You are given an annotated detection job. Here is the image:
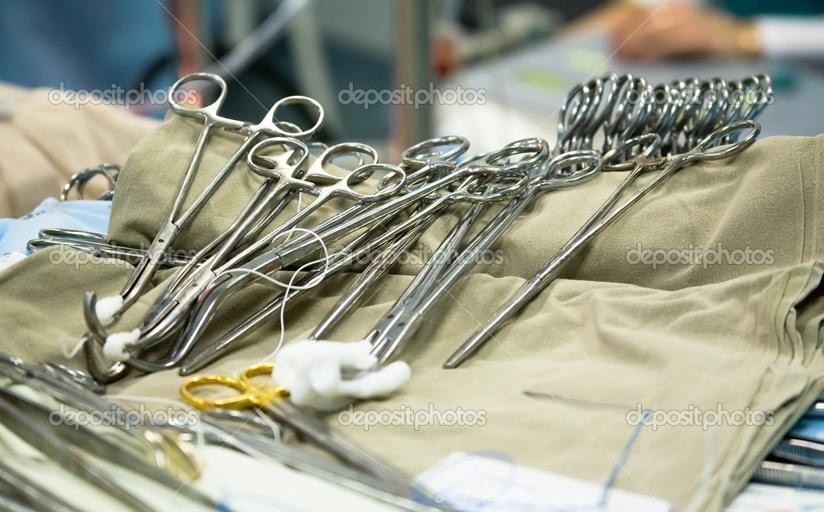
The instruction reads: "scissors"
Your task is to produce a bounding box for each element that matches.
[60,66,775,388]
[0,349,470,512]
